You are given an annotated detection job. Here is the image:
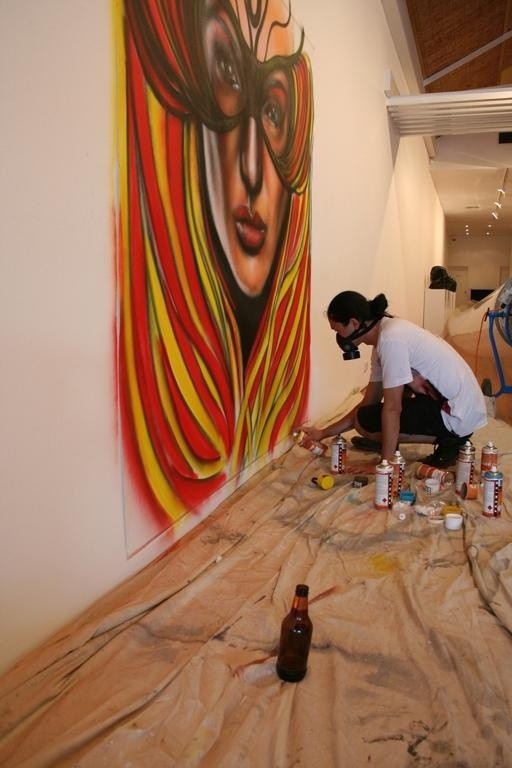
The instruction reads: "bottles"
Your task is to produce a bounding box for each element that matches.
[273,583,315,686]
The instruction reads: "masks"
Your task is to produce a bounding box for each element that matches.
[336,330,361,352]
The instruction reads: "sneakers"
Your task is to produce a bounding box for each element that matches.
[351,436,400,451]
[424,434,473,468]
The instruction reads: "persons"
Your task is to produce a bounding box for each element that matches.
[111,0,311,534]
[289,290,489,470]
